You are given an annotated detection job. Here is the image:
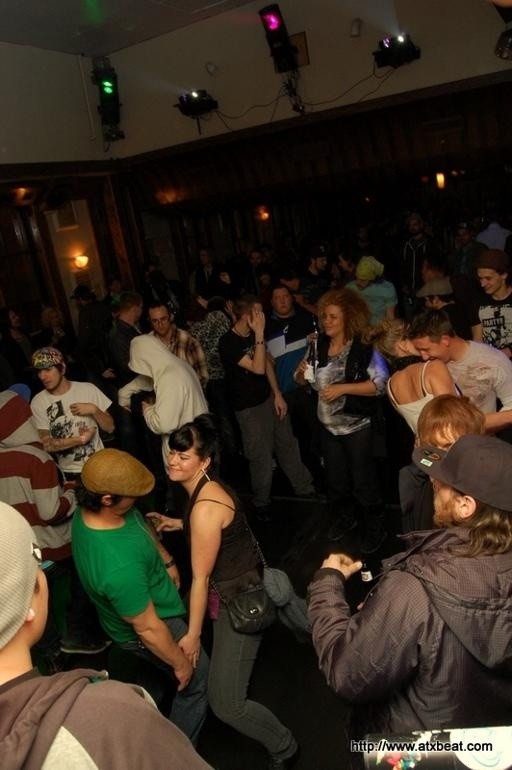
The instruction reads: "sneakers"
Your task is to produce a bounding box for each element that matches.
[360,528,388,553]
[328,513,357,540]
[267,742,301,770]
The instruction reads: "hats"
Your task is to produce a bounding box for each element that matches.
[81,448,155,497]
[0,503,34,651]
[31,347,62,369]
[475,249,509,274]
[416,277,453,298]
[413,433,512,513]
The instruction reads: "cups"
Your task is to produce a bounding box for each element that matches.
[149,518,163,540]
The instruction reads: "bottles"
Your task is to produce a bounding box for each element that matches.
[304,343,319,384]
[312,320,319,342]
[360,560,375,592]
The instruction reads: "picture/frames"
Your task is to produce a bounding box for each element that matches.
[49,204,81,233]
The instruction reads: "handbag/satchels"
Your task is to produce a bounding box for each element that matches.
[224,585,278,633]
